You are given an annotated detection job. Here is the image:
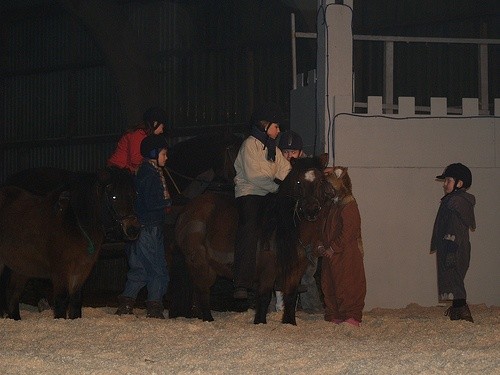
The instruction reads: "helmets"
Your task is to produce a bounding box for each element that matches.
[279,131,303,151]
[140,135,168,157]
[436,164,472,189]
[255,102,285,123]
[143,108,169,125]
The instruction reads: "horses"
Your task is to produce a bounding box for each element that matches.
[0,176,143,320]
[175,154,329,327]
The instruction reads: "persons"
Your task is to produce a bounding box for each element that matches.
[106,106,168,169]
[430,163,476,321]
[234,119,292,298]
[116,136,175,318]
[275,131,321,312]
[317,167,366,326]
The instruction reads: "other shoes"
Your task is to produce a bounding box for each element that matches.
[443,306,473,323]
[341,319,358,327]
[116,304,132,315]
[331,319,339,325]
[234,288,248,299]
[147,304,164,319]
